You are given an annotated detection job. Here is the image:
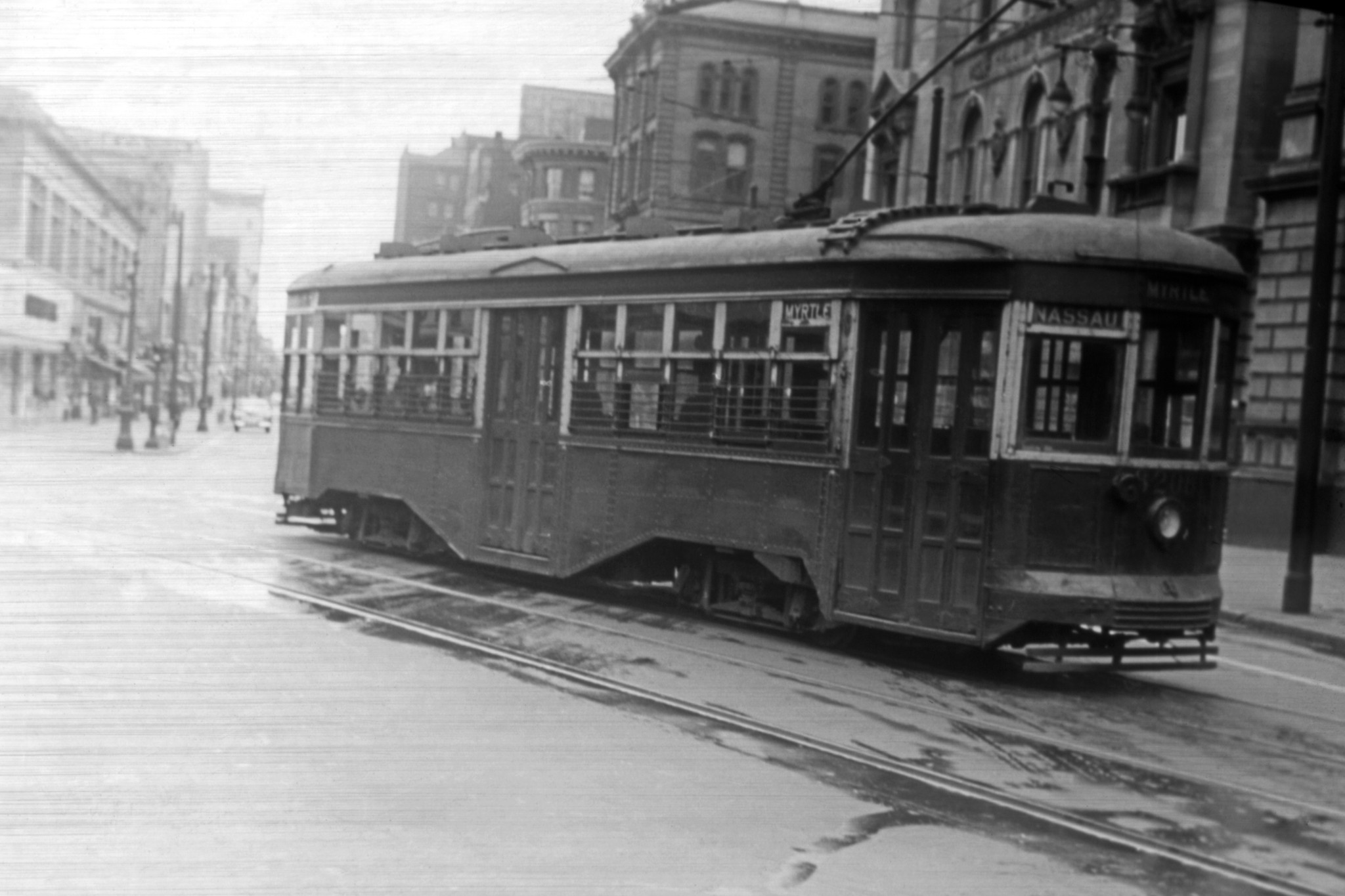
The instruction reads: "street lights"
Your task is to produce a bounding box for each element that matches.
[1047,42,1164,210]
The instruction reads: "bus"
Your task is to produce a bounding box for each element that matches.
[273,201,1258,676]
